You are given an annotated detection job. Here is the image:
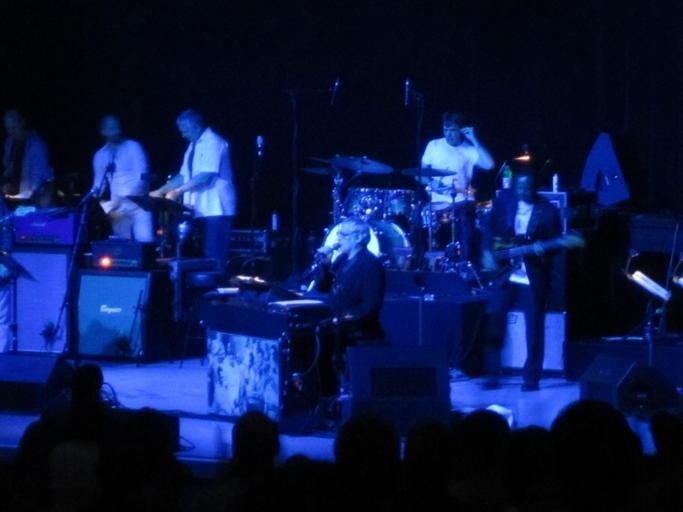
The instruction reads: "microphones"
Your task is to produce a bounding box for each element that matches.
[312,243,341,263]
[255,136,265,156]
[329,79,341,105]
[108,143,117,175]
[401,78,411,107]
[204,286,239,298]
[178,204,194,239]
[77,184,98,207]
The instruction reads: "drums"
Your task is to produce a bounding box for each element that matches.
[420,201,451,229]
[346,187,388,222]
[321,223,411,279]
[381,188,417,218]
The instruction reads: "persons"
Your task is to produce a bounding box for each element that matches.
[149,108,237,274]
[86,114,153,242]
[479,172,561,393]
[421,110,498,258]
[0,108,57,209]
[1,398,682,512]
[299,219,386,396]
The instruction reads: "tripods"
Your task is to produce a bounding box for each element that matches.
[415,178,485,293]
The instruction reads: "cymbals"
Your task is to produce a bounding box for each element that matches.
[334,155,393,174]
[402,168,456,178]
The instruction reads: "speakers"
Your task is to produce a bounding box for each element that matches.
[11,245,76,356]
[614,360,682,420]
[73,267,172,363]
[0,350,76,411]
[88,238,155,271]
[12,207,86,245]
[341,346,452,428]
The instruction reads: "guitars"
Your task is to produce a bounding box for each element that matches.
[476,230,587,279]
